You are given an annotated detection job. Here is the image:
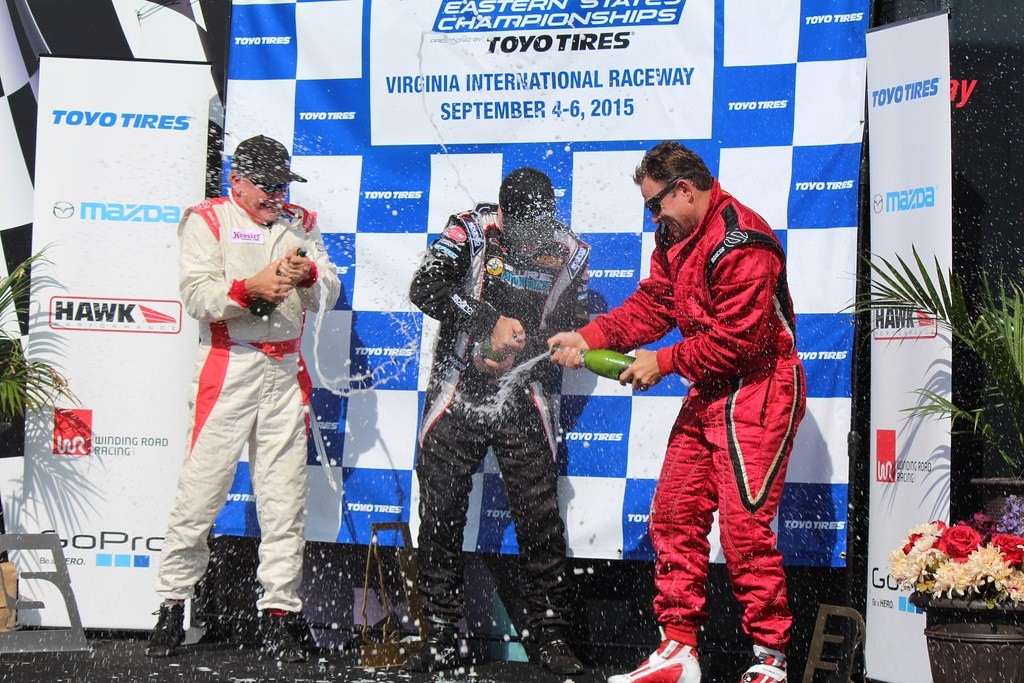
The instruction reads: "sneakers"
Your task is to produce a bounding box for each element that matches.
[528,625,584,675]
[400,623,462,670]
[258,613,305,662]
[741,643,788,683]
[605,626,701,683]
[148,605,185,656]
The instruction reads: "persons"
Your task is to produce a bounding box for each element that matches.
[143,134,342,661]
[410,169,591,676]
[546,140,807,683]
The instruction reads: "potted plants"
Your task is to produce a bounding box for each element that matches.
[837,243,1024,531]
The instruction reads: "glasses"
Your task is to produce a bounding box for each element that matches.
[645,175,684,216]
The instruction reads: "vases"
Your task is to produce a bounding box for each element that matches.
[907,589,1023,682]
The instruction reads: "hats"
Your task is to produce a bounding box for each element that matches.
[499,168,555,243]
[230,135,308,192]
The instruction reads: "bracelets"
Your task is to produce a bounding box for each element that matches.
[641,386,646,391]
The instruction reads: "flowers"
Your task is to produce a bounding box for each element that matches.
[887,495,1023,610]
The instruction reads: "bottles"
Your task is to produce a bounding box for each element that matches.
[249,248,307,317]
[551,346,637,384]
[471,334,523,363]
